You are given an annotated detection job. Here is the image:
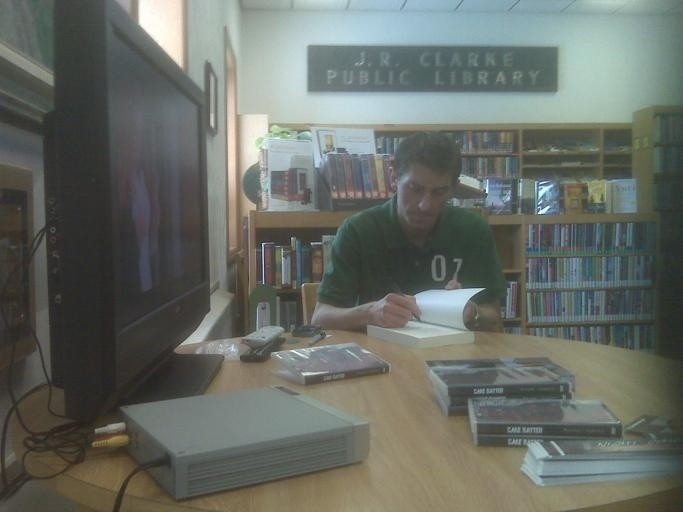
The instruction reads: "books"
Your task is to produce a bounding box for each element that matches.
[366,288,485,347]
[447,129,519,215]
[261,136,405,211]
[258,235,335,329]
[654,115,681,177]
[522,140,626,153]
[501,280,521,335]
[519,179,655,352]
[423,353,628,449]
[272,342,391,386]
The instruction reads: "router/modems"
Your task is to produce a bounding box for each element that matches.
[117,384,370,500]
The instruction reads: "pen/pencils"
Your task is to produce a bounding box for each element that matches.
[310,332,326,345]
[393,283,422,322]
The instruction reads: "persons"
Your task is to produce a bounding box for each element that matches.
[309,133,509,332]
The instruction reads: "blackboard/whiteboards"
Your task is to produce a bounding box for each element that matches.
[308,45,557,92]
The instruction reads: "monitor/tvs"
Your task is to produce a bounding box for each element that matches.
[43,1,223,421]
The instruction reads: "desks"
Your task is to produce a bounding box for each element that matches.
[24,328,683,512]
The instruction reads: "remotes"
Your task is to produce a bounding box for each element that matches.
[240,325,283,348]
[241,337,286,362]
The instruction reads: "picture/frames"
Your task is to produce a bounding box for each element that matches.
[201,62,220,135]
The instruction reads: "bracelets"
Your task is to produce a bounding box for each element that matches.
[472,306,479,327]
